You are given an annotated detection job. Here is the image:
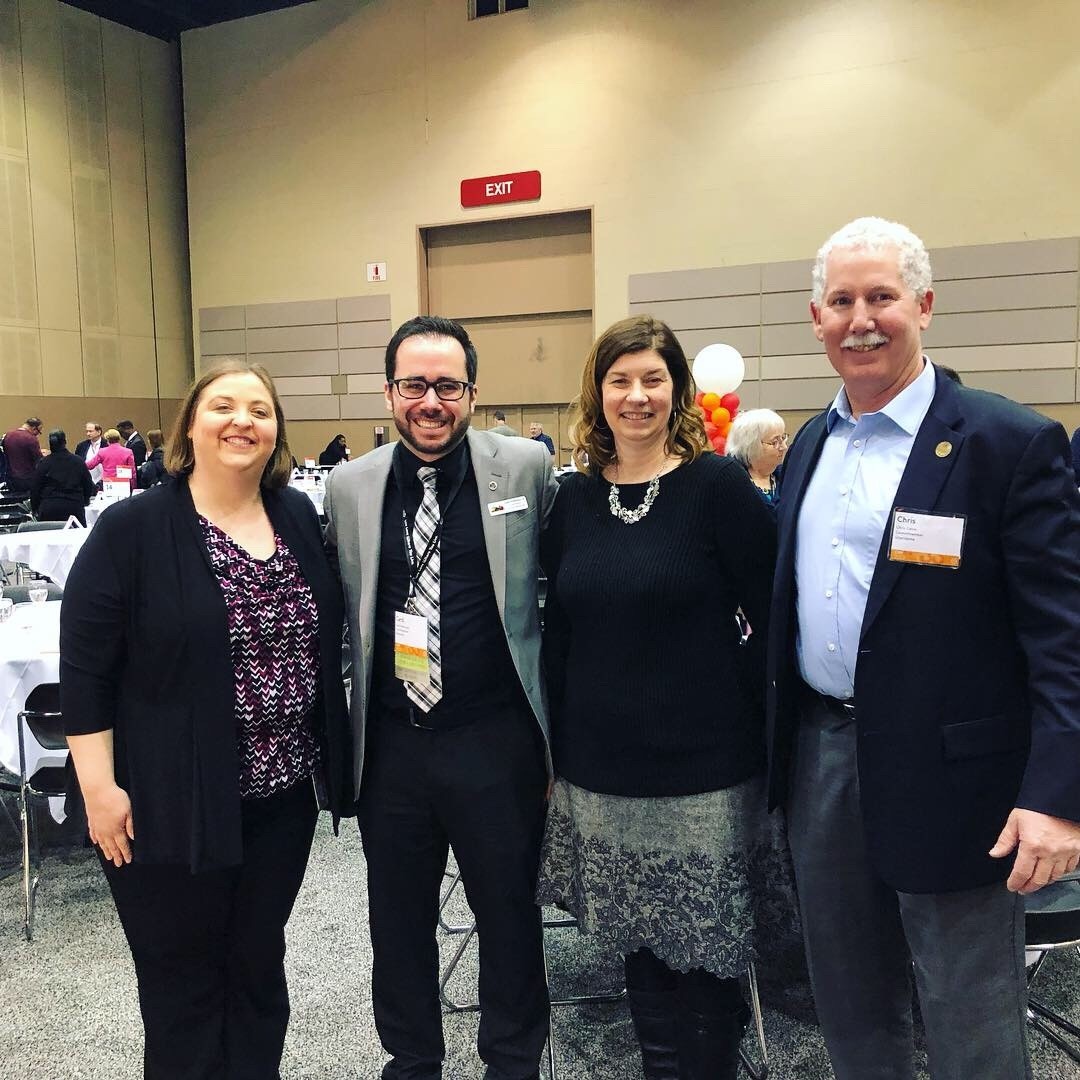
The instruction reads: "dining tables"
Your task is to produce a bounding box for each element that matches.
[0,600,72,792]
[85,499,126,526]
[291,480,328,514]
[0,526,96,590]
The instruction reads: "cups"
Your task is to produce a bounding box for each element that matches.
[0,598,13,624]
[29,590,48,606]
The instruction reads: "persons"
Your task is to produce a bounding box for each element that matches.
[533,313,803,1080]
[58,360,355,1080]
[116,421,147,467]
[489,411,517,437]
[146,429,167,473]
[529,423,555,462]
[723,409,788,506]
[325,316,562,1080]
[3,417,45,494]
[85,428,137,490]
[319,434,353,470]
[31,430,92,529]
[76,423,109,491]
[759,217,1080,1080]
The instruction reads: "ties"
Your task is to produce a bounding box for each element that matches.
[406,467,441,713]
[92,442,98,455]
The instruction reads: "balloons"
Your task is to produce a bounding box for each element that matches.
[691,344,744,399]
[693,393,740,456]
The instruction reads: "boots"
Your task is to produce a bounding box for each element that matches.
[631,999,741,1079]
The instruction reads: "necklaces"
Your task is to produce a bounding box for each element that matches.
[608,455,668,524]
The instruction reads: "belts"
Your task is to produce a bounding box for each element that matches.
[799,682,855,718]
[377,705,498,730]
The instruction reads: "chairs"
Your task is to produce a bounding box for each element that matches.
[17,683,82,943]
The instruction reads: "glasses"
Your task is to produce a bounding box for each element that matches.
[389,378,473,402]
[36,429,42,435]
[762,433,790,448]
